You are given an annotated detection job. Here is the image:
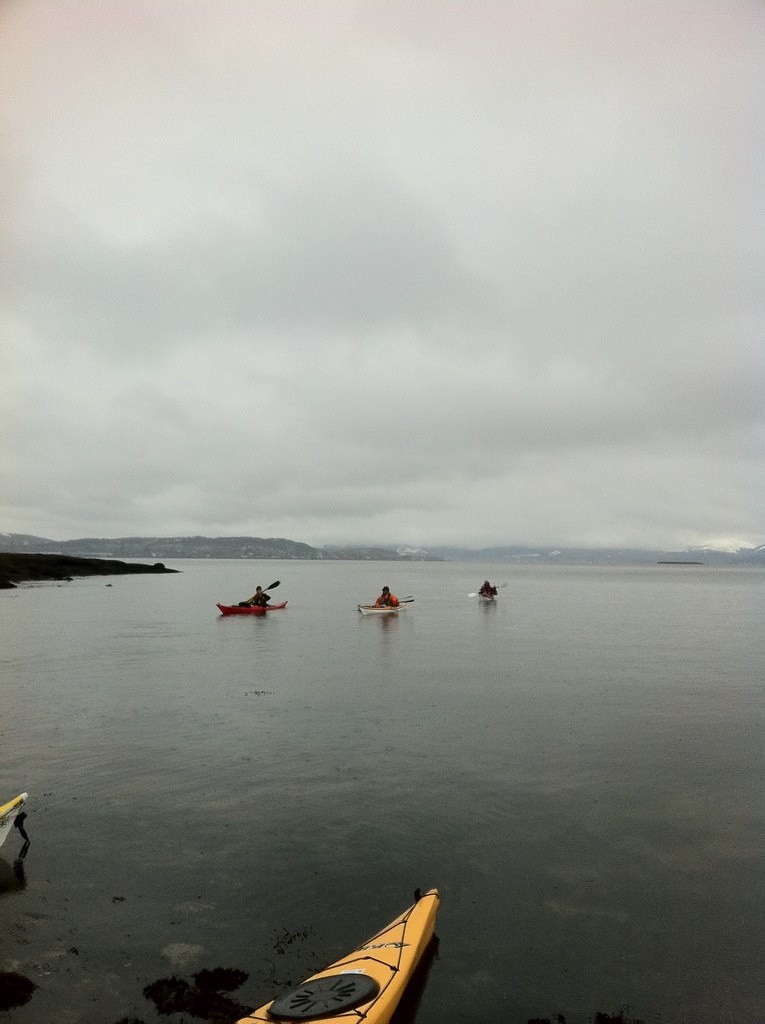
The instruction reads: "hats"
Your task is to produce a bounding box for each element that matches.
[382,586,389,591]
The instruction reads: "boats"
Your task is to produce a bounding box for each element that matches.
[235,887,440,1024]
[0,792,30,848]
[358,604,407,615]
[479,589,499,602]
[216,601,288,615]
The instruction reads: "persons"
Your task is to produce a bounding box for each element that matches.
[375,586,399,606]
[480,581,497,595]
[252,586,271,606]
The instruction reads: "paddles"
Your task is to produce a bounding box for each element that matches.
[357,599,415,612]
[246,581,280,602]
